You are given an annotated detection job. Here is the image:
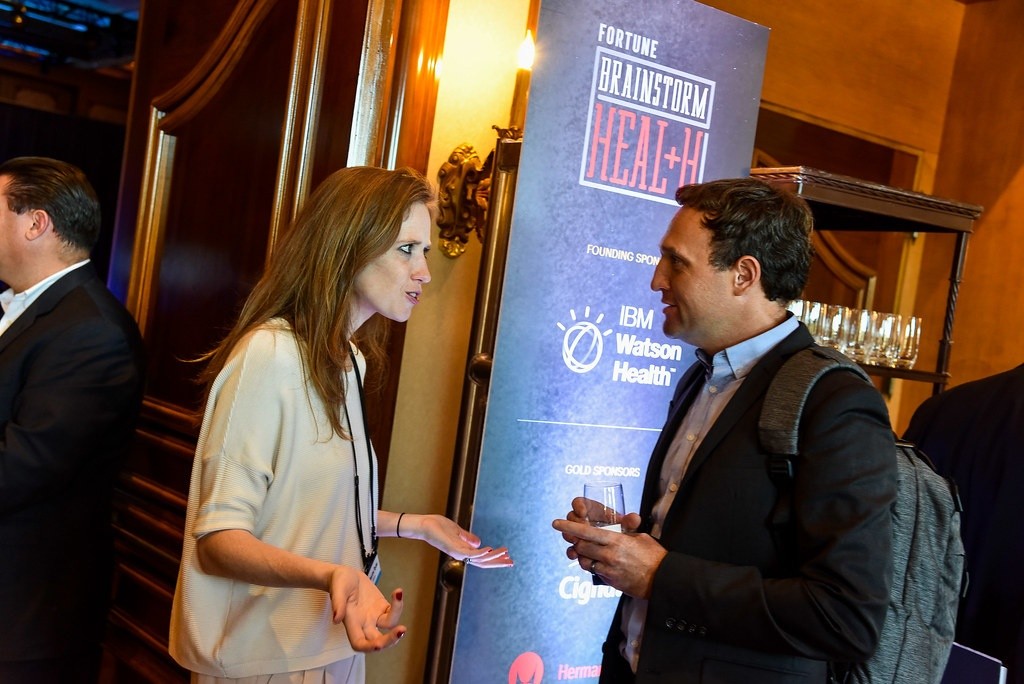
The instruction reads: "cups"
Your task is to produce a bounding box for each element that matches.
[584,481,628,534]
[784,298,923,370]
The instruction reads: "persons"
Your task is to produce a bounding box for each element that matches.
[0,157,151,684]
[900,364,1024,684]
[169,165,515,684]
[552,177,901,684]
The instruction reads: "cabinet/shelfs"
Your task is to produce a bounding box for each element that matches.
[750,165,985,397]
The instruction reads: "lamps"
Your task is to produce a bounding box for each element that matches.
[435,28,537,259]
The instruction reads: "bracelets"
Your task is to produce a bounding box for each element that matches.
[396,513,406,538]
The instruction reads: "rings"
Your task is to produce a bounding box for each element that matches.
[590,560,599,573]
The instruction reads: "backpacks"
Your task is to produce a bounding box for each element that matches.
[759,343,967,684]
